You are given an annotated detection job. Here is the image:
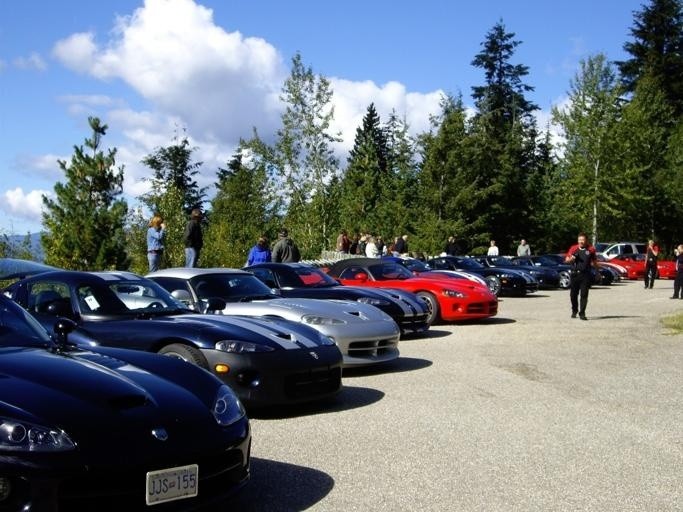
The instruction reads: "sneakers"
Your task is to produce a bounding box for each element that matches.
[670,296,679,299]
[571,310,577,318]
[644,285,648,289]
[579,312,587,320]
[650,285,653,289]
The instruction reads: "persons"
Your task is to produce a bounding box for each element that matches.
[564,232,597,320]
[146,215,166,272]
[445,237,457,256]
[269,227,300,264]
[674,246,680,272]
[670,245,683,299]
[246,236,271,267]
[486,240,499,256]
[181,209,202,268]
[337,224,409,261]
[643,240,659,289]
[517,239,531,256]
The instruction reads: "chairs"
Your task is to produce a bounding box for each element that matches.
[35,290,61,306]
